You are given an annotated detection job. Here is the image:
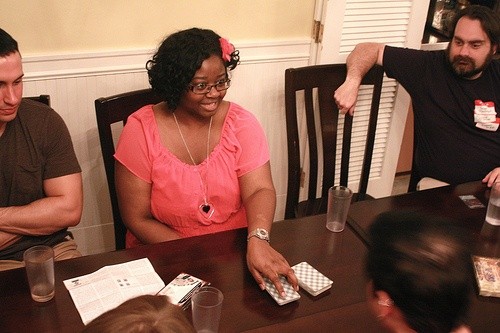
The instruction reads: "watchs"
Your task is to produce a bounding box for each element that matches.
[246,227,270,244]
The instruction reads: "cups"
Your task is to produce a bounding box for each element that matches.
[23,245,55,303]
[485,182,500,226]
[326,186,353,232]
[191,287,224,333]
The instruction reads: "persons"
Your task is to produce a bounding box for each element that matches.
[0,27,84,271]
[112,29,299,297]
[363,206,474,333]
[332,6,500,191]
[79,295,196,333]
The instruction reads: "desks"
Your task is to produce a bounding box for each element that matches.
[0,181,500,333]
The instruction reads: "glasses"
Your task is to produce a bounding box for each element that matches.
[186,78,231,95]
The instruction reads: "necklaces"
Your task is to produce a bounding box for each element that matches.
[170,108,214,219]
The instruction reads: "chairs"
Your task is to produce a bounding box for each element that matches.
[95,89,166,250]
[285,64,384,220]
